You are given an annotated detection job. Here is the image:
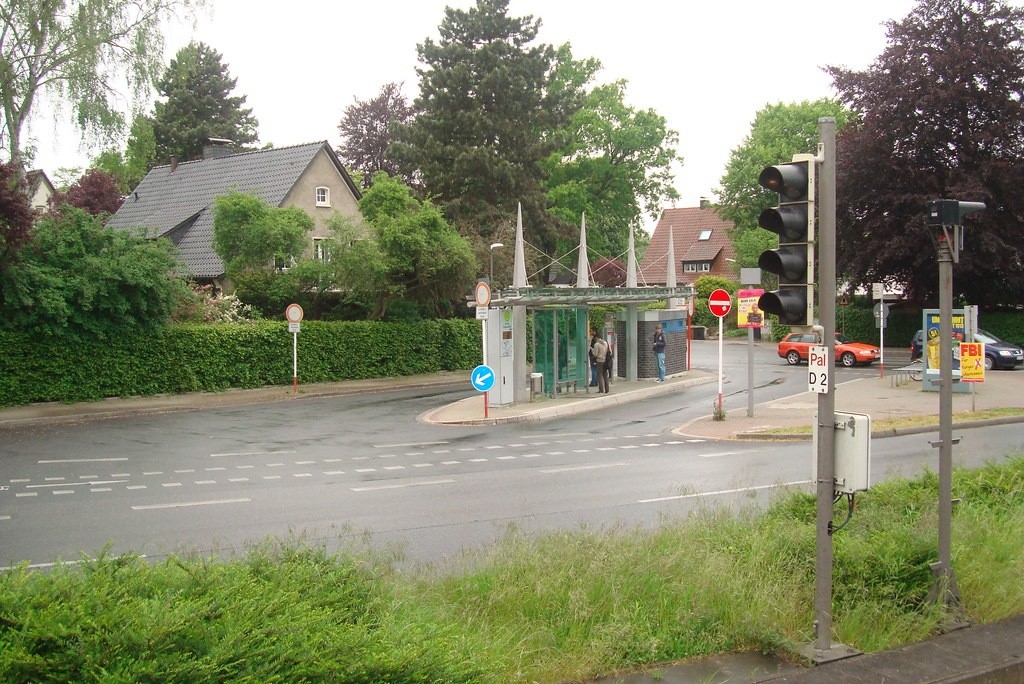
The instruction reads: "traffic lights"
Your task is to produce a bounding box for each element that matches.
[757,154,815,326]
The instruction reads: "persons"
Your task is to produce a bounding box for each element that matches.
[558,329,567,393]
[652,324,666,383]
[747,304,763,321]
[590,334,612,394]
[589,326,602,387]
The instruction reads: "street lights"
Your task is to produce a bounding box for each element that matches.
[489,242,504,284]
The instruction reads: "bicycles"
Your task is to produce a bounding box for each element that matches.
[908,358,923,381]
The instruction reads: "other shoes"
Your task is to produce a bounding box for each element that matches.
[656,378,664,383]
[595,391,604,394]
[589,383,598,387]
[605,391,609,394]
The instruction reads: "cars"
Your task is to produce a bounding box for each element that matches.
[777,331,881,367]
[911,327,1024,370]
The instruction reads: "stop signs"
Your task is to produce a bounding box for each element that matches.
[708,289,731,317]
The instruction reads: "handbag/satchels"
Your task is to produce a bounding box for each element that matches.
[604,340,612,369]
